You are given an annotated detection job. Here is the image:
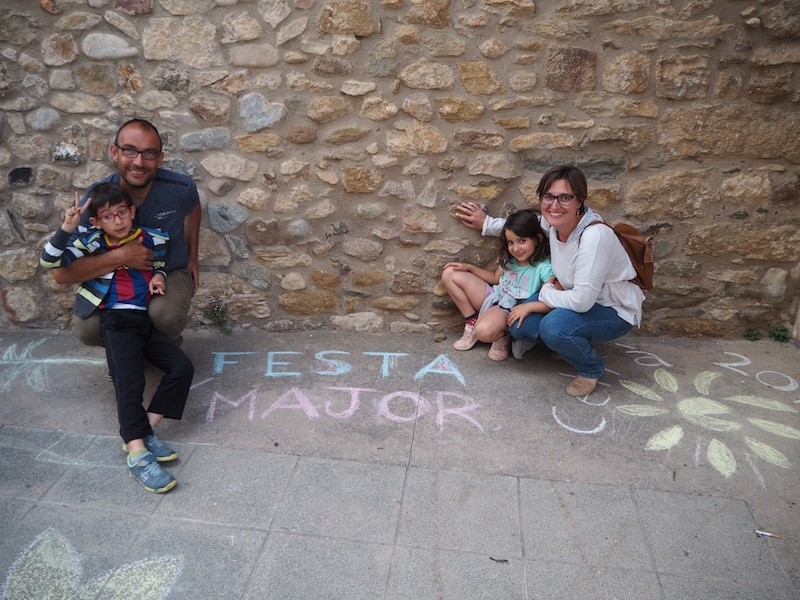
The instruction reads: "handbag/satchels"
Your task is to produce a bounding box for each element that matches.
[577,221,654,290]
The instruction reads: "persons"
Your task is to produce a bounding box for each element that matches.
[52,119,202,381]
[441,210,562,362]
[39,181,194,493]
[455,165,646,396]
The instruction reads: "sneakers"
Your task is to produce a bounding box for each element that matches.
[126,452,178,494]
[488,335,511,361]
[123,431,178,462]
[454,322,479,351]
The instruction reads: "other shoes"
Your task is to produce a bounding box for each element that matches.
[107,369,113,381]
[565,375,598,397]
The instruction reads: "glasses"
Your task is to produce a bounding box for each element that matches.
[540,193,575,205]
[115,142,161,160]
[95,205,131,224]
